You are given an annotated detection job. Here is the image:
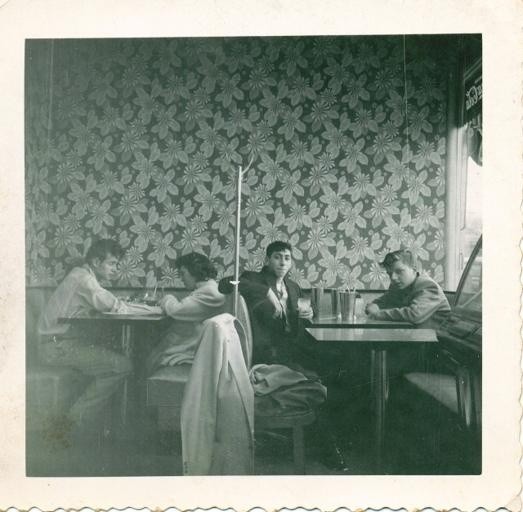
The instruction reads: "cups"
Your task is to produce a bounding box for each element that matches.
[310,286,324,314]
[331,288,356,322]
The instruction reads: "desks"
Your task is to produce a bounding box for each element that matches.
[55,306,169,475]
[305,309,438,473]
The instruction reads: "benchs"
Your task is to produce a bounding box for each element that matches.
[147,290,316,476]
[24,294,84,475]
[401,360,481,475]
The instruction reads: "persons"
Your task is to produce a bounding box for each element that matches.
[219,242,355,472]
[363,249,456,375]
[134,253,232,449]
[33,238,135,451]
